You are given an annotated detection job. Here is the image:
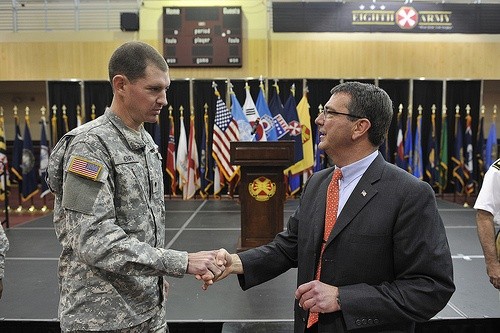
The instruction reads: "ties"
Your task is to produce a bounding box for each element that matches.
[307,168,342,328]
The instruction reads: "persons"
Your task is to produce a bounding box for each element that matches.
[473,158,500,290]
[44,40,227,333]
[203,82,456,333]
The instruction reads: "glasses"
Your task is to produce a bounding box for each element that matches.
[324,108,364,120]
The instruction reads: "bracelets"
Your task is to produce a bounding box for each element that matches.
[336,294,341,306]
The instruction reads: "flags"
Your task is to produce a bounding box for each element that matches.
[380,114,497,197]
[207,82,333,198]
[151,111,213,201]
[0,113,95,201]
